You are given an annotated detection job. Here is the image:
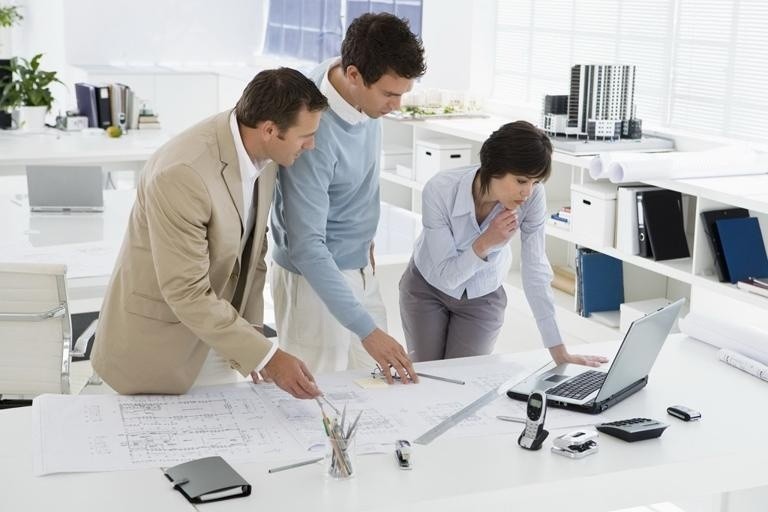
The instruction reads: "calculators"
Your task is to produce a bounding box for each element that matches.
[596,418,668,441]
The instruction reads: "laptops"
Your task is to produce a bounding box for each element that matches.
[505,295,687,415]
[25,164,106,213]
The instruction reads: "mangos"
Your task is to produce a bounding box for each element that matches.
[107,126,122,137]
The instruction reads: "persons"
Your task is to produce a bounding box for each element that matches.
[268,12,427,386]
[86,64,329,400]
[397,119,610,368]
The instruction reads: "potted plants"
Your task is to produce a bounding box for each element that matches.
[0,51,70,133]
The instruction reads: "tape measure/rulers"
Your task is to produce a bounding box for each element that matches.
[413,356,554,446]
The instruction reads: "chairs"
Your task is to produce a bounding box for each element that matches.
[0,260,98,400]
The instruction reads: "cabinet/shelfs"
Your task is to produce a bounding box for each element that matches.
[376,111,767,332]
[72,60,259,128]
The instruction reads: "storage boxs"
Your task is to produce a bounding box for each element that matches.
[380,137,471,186]
[569,182,688,247]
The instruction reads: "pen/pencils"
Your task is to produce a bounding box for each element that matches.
[316,395,342,417]
[416,372,465,385]
[269,458,326,473]
[322,404,364,477]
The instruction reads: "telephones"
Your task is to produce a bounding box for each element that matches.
[518,391,548,451]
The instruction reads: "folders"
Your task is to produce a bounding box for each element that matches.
[636,190,689,257]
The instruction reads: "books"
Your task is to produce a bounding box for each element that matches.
[164,455,251,505]
[75,82,161,133]
[616,184,768,299]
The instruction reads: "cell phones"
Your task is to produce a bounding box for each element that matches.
[667,405,702,422]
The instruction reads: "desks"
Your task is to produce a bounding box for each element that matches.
[1,333,766,512]
